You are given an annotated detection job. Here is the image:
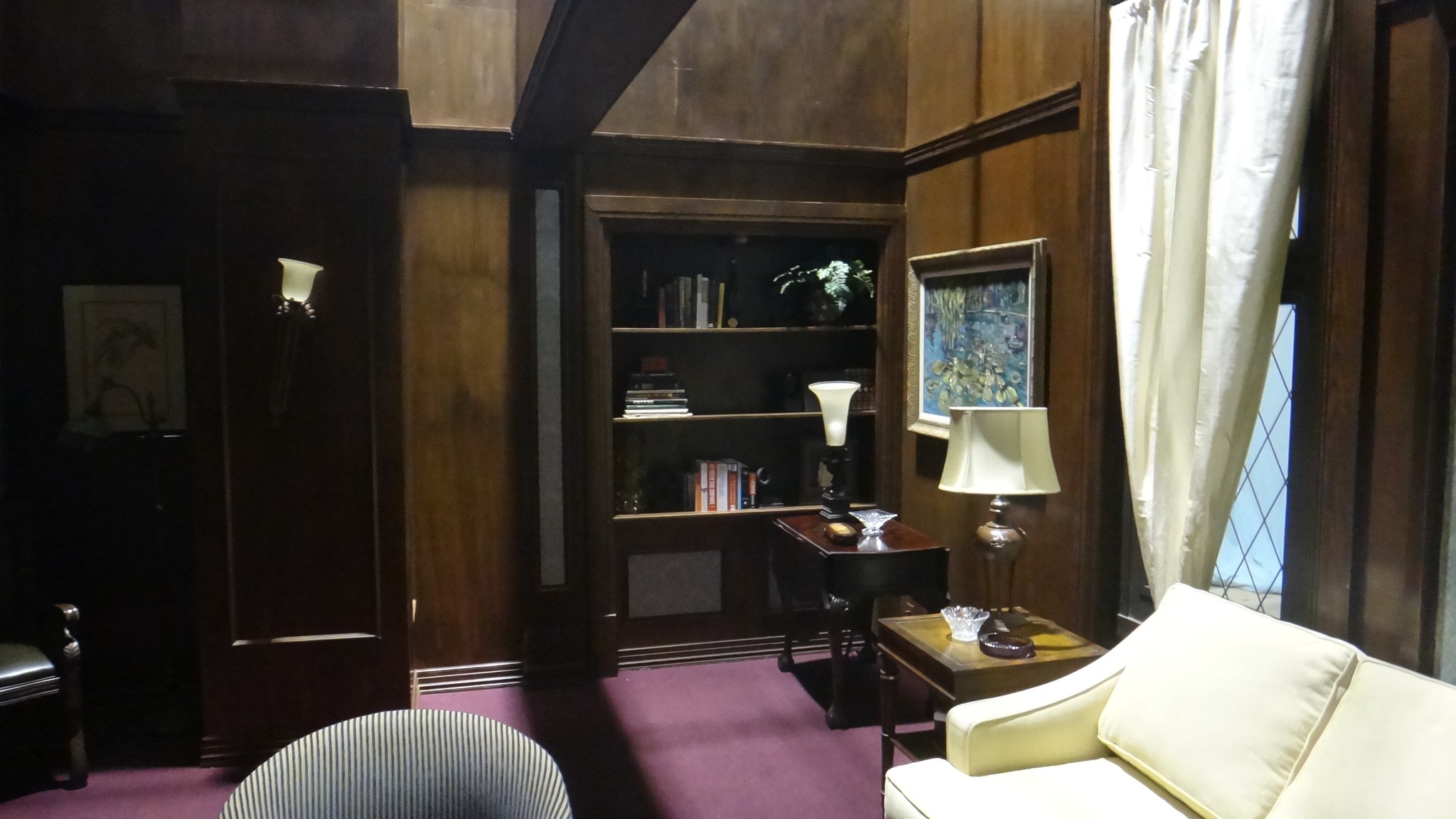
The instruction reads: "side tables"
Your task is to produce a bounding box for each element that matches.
[877,606,1111,813]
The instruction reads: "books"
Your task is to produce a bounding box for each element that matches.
[619,352,693,417]
[657,272,725,329]
[681,456,756,513]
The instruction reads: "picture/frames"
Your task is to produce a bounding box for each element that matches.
[63,285,187,437]
[905,236,1048,443]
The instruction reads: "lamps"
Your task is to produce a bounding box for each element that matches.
[937,405,1062,635]
[806,380,862,522]
[266,254,324,424]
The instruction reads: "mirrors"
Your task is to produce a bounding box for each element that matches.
[218,147,387,647]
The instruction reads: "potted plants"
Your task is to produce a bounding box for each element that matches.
[773,251,875,325]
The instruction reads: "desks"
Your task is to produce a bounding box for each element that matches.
[770,512,950,730]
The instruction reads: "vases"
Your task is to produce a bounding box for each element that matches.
[619,434,650,513]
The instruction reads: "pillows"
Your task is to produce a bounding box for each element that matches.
[1265,658,1456,819]
[1098,583,1358,819]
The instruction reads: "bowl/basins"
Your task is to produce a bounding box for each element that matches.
[941,605,990,641]
[849,509,898,535]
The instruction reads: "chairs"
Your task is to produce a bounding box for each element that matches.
[0,595,90,793]
[218,707,575,819]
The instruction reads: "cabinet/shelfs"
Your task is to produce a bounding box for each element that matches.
[584,193,911,664]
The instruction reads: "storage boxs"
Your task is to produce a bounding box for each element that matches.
[825,522,859,545]
[636,355,667,373]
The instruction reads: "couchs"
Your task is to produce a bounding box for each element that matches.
[884,583,1456,819]
